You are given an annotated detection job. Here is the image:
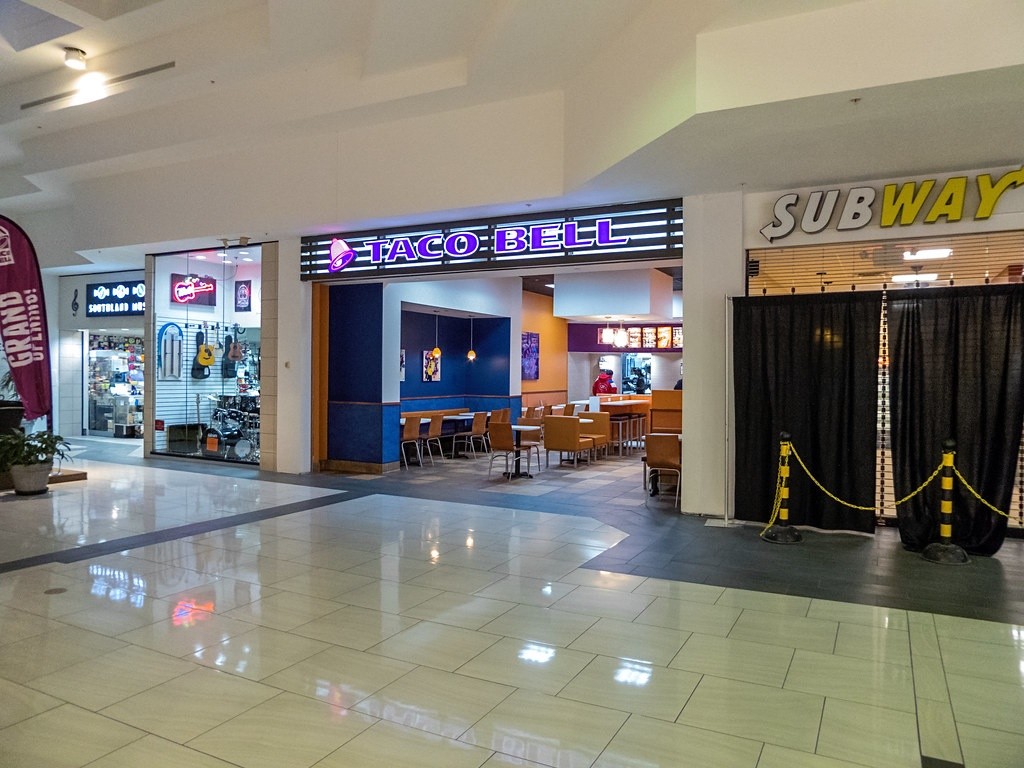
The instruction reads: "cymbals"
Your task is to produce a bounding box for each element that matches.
[204,392,221,402]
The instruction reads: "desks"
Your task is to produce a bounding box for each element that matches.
[400,418,431,466]
[503,425,540,479]
[561,419,593,465]
[442,416,473,459]
[599,399,649,405]
[459,411,492,417]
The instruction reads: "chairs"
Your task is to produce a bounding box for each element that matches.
[400,393,682,509]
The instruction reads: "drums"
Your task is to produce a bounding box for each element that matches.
[211,407,226,422]
[234,438,256,459]
[218,394,239,410]
[241,413,260,432]
[239,395,260,414]
[226,409,243,425]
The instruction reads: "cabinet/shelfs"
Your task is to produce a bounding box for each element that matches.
[113,395,144,438]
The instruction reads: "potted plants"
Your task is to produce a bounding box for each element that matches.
[0,428,73,496]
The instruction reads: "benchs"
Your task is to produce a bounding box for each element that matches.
[400,408,470,454]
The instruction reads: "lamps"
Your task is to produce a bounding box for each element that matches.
[433,309,442,358]
[467,315,476,361]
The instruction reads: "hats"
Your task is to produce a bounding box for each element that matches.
[642,365,651,369]
[634,367,642,371]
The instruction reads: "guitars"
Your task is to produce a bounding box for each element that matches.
[194,394,203,449]
[197,320,217,366]
[229,324,242,360]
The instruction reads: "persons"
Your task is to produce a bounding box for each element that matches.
[592,370,617,397]
[131,384,142,395]
[673,378,682,391]
[634,368,646,394]
